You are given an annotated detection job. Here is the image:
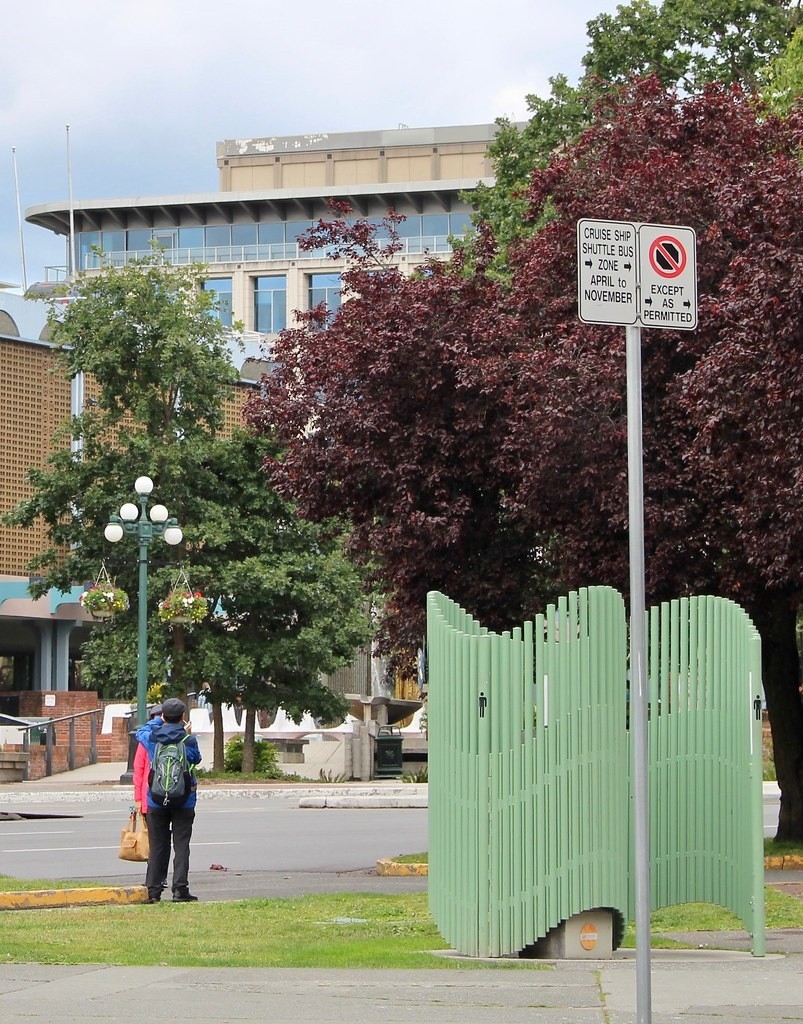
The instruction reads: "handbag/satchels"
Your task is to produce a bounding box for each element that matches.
[118,810,149,861]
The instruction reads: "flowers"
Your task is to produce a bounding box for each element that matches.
[156,588,207,633]
[79,583,130,622]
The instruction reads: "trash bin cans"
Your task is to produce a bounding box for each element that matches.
[376,724,405,775]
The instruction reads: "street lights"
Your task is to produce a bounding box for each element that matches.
[103,476,184,786]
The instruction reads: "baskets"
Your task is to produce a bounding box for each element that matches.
[92,567,115,616]
[168,572,194,623]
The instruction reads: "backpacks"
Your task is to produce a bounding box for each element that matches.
[148,734,195,806]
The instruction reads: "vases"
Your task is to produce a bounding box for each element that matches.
[92,609,115,617]
[170,616,192,623]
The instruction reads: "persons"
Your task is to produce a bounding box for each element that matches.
[135,698,202,904]
[132,705,172,888]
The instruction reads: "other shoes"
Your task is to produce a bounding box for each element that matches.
[173,894,198,903]
[143,897,160,904]
[160,879,168,888]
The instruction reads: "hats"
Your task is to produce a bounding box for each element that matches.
[150,704,162,716]
[162,698,185,716]
